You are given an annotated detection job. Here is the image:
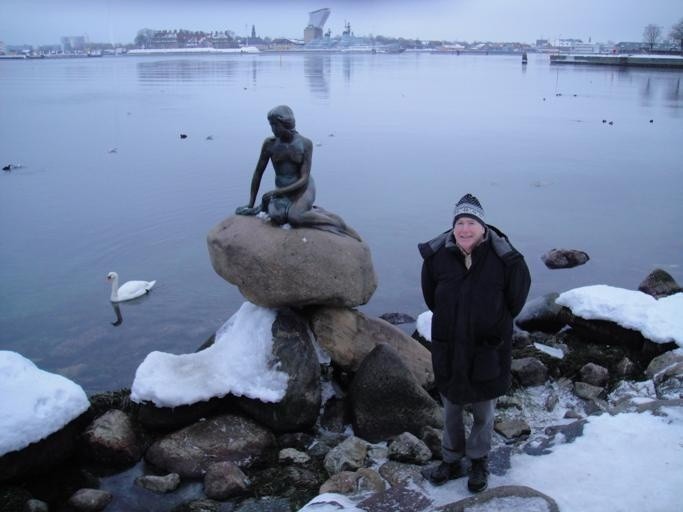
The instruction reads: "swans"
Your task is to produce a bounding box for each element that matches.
[108,291,155,328]
[104,271,157,305]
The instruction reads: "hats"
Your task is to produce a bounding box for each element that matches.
[452,192,486,229]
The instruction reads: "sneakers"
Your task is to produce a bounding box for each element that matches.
[468,456,488,492]
[429,460,461,486]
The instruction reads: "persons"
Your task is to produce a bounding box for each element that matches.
[235,106,362,238]
[417,193,531,494]
